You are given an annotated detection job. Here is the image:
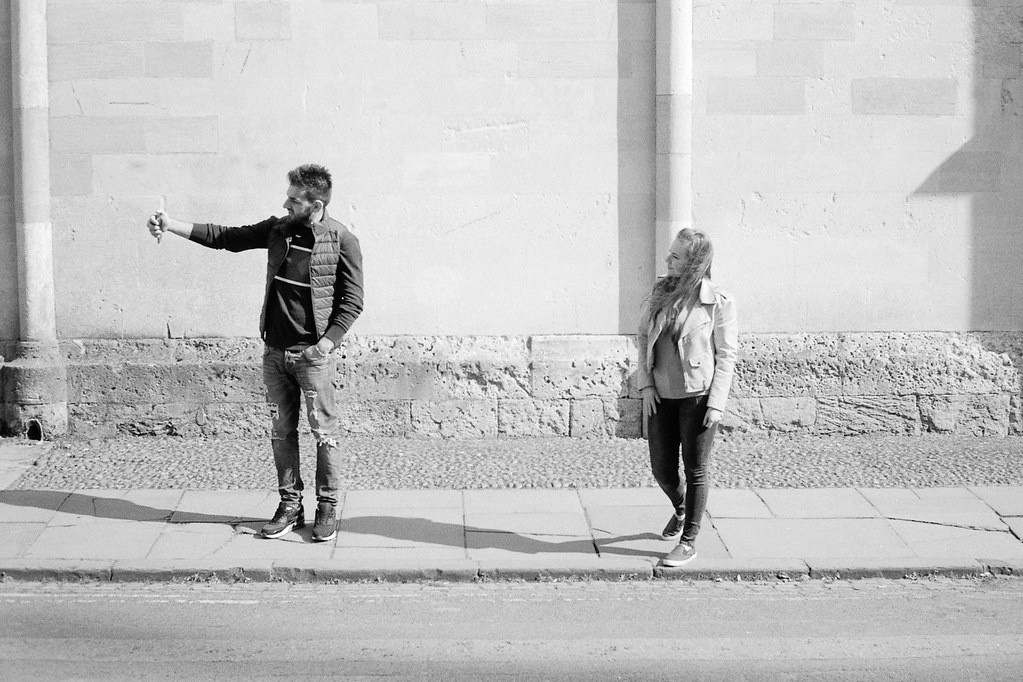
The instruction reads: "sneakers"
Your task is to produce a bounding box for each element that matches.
[261,503,304,538]
[312,503,337,541]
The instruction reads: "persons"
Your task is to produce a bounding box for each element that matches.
[633,227,737,567]
[147,164,364,542]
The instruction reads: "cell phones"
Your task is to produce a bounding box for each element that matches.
[155,213,163,243]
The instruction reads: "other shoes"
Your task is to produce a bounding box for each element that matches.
[663,545,697,567]
[662,514,686,539]
